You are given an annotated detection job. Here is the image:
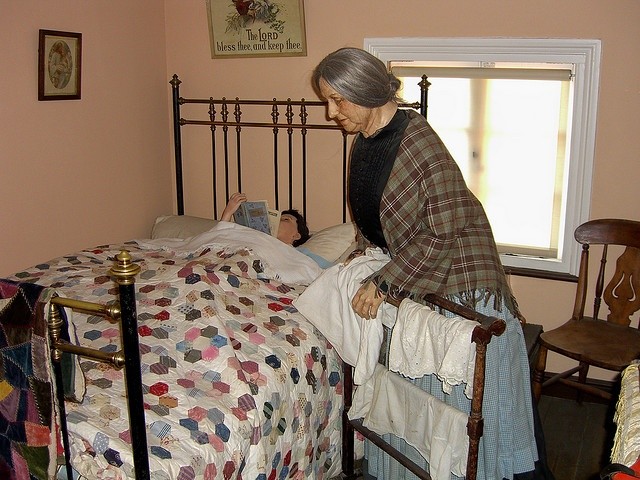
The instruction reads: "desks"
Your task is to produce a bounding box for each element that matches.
[521,323,544,361]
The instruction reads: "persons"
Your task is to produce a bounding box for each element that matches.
[217,189,310,247]
[312,48,539,480]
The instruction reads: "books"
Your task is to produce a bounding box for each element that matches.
[232,198,280,241]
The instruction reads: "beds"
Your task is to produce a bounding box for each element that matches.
[0,72,432,480]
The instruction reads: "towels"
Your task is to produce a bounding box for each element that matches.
[0,278,86,480]
[346,364,471,478]
[291,248,399,385]
[389,298,481,400]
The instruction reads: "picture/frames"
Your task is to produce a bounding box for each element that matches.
[37,30,82,102]
[205,1,308,59]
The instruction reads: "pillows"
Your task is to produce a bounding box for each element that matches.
[610,358,640,473]
[151,215,358,267]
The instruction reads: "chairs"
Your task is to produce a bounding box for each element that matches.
[532,218,640,404]
